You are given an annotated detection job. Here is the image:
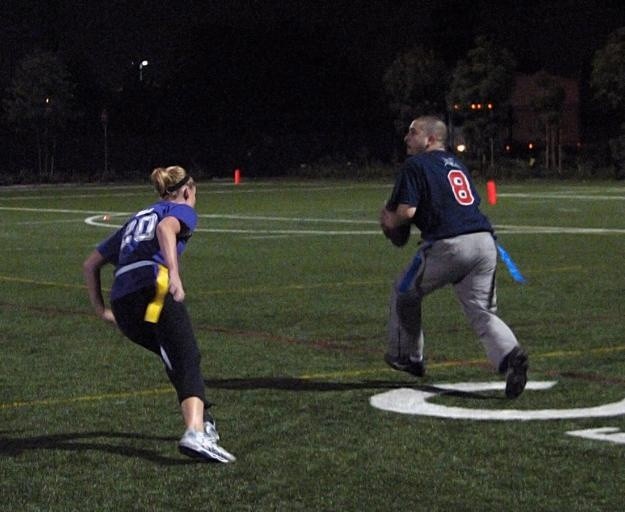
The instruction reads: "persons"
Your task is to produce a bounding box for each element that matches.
[82,164,240,466]
[378,114,533,400]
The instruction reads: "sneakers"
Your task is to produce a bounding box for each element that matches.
[177,428,237,464]
[506,353,529,399]
[383,351,426,377]
[203,420,222,443]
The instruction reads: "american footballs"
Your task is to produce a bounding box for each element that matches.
[392,222,412,246]
